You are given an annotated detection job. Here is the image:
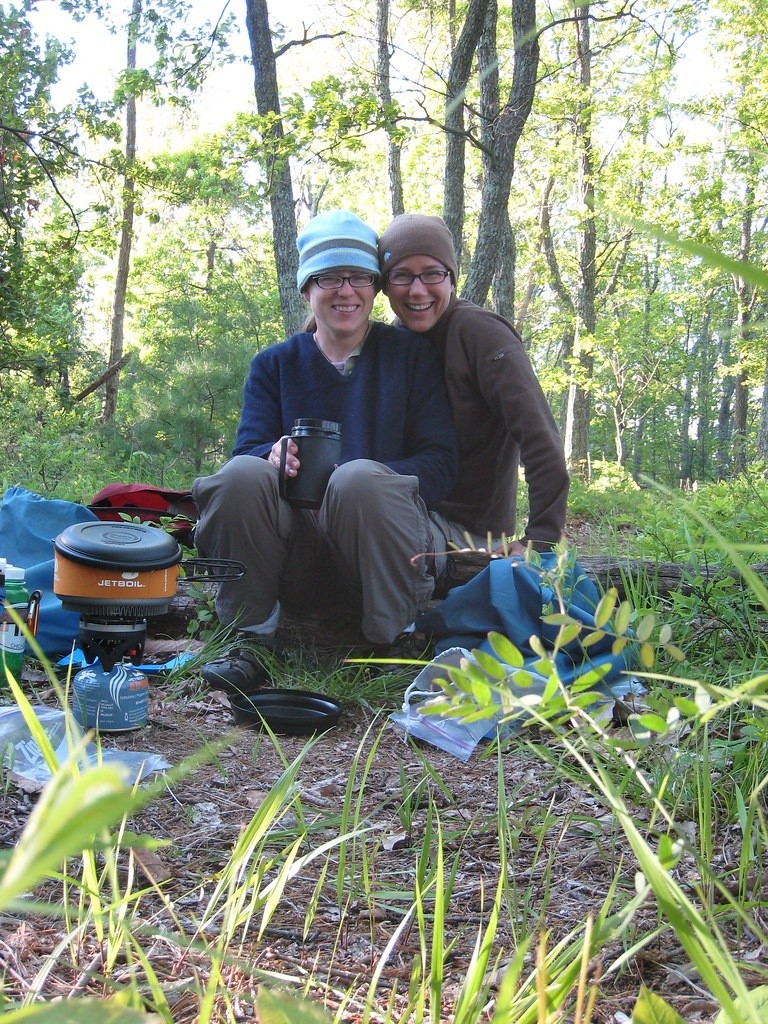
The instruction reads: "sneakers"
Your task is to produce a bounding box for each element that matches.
[375,634,423,683]
[200,636,278,693]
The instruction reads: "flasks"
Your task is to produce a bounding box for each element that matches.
[279,418,343,510]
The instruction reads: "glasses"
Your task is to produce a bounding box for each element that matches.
[384,270,452,286]
[311,272,379,289]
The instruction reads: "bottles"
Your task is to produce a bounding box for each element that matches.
[0,568,43,688]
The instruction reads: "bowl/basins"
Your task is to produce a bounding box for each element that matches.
[232,690,342,734]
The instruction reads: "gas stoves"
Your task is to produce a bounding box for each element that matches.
[71,611,148,733]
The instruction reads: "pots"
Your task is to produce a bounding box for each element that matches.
[52,521,248,607]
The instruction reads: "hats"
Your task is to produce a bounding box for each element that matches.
[377,214,458,289]
[295,210,383,295]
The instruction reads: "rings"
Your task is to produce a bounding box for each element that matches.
[275,457,279,465]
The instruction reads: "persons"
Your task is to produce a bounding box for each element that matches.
[191,210,462,691]
[378,214,570,580]
